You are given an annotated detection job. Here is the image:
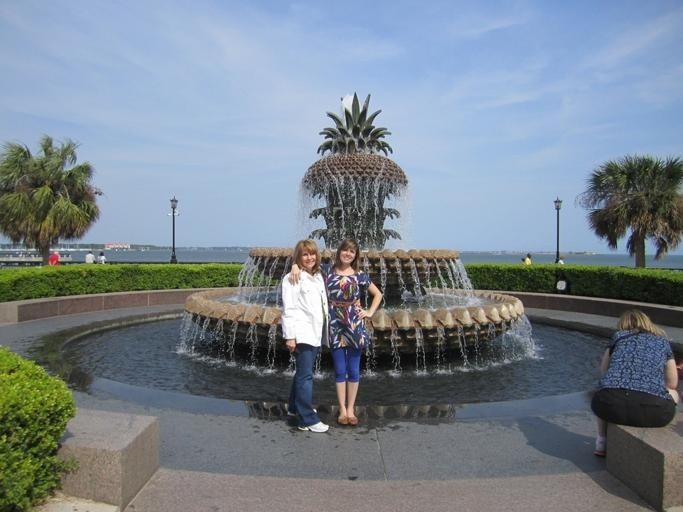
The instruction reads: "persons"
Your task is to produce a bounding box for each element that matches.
[591,309,678,457]
[523,253,532,265]
[86,251,96,264]
[558,256,565,264]
[48,251,59,266]
[289,238,382,426]
[97,251,106,264]
[281,240,330,432]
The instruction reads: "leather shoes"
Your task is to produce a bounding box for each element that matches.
[338,416,358,425]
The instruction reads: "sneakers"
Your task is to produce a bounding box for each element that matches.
[288,409,329,432]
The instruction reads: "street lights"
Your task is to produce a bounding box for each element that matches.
[553,197,562,263]
[170,195,177,264]
